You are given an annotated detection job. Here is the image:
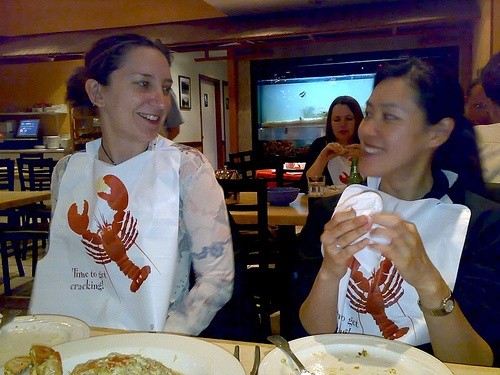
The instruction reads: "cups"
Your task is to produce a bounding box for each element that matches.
[307,175,326,197]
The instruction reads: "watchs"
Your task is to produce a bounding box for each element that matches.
[417,291,455,317]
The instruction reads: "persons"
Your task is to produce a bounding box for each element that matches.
[464,53,500,202]
[28,33,235,338]
[299,97,365,193]
[278,52,500,369]
[164,90,184,141]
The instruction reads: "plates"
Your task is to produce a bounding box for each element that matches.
[34,146,45,149]
[256,334,453,375]
[52,332,248,375]
[43,135,60,148]
[0,313,91,375]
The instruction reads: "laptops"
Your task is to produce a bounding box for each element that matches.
[5,118,42,141]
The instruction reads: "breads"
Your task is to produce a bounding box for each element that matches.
[70,352,174,375]
[4,345,64,375]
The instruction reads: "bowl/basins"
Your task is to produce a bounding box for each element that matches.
[61,138,70,148]
[267,187,301,206]
[32,108,43,112]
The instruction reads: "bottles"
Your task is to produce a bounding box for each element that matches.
[346,162,365,187]
[8,130,14,137]
[0,133,5,143]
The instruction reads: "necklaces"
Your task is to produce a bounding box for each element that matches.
[101,139,115,165]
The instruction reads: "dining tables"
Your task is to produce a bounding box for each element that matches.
[228,189,309,341]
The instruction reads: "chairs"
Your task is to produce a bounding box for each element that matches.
[0,147,342,345]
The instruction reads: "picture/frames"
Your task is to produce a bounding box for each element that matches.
[178,75,192,110]
